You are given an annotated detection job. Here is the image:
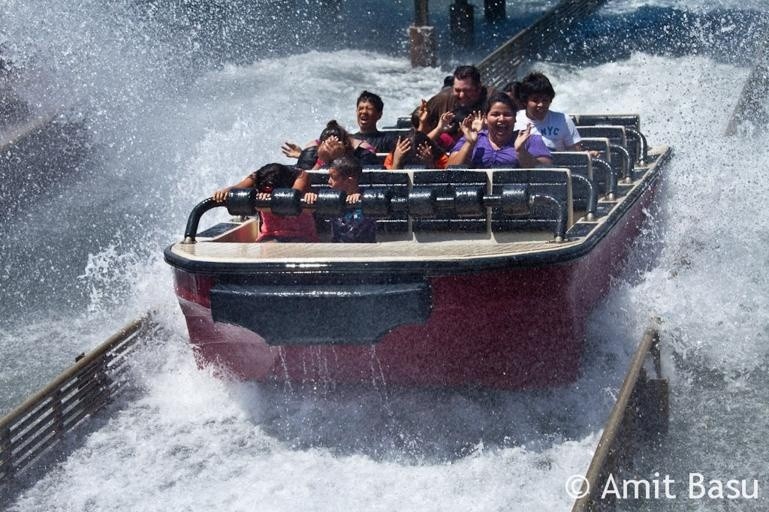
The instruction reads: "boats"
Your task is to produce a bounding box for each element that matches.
[162,112,676,389]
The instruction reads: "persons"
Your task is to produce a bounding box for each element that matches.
[212,65,593,244]
